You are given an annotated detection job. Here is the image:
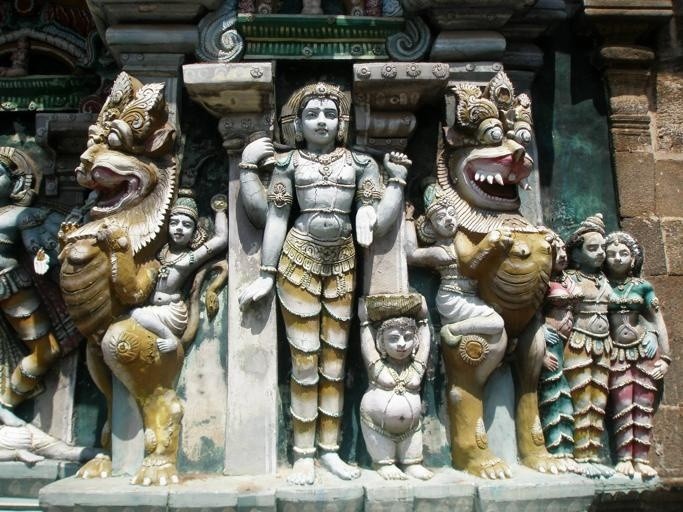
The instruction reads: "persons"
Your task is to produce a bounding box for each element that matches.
[358,292,436,481]
[134,189,228,353]
[238,83,412,485]
[406,184,504,344]
[538,214,672,478]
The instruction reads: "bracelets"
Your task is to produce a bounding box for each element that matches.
[388,178,406,185]
[238,162,258,170]
[418,318,429,325]
[360,320,373,327]
[259,265,277,274]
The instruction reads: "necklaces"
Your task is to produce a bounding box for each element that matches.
[157,243,187,278]
[298,148,346,180]
